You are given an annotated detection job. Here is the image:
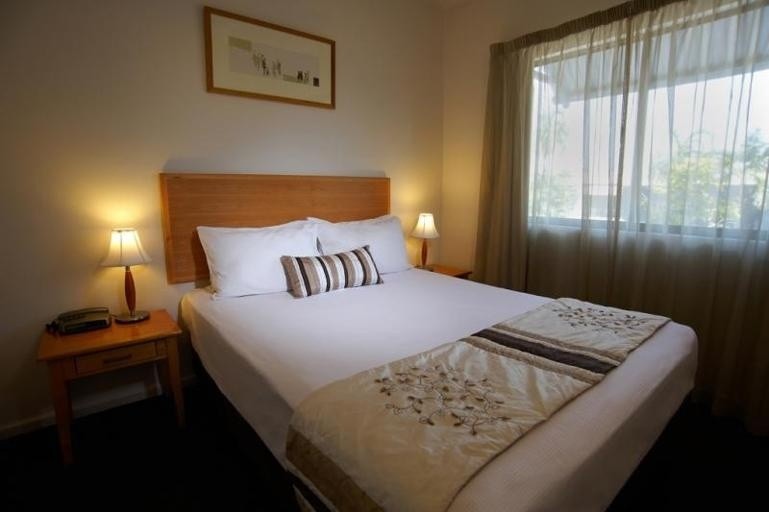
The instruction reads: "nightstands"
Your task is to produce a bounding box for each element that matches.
[36,309,184,464]
[424,264,473,282]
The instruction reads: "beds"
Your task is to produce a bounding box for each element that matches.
[162,171,699,512]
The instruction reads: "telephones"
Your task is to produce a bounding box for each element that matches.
[46,306,111,335]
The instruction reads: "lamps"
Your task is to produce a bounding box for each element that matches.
[411,213,440,272]
[101,229,152,325]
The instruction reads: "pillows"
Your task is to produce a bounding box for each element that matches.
[309,215,411,274]
[196,219,320,297]
[281,245,385,298]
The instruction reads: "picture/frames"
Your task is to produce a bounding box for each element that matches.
[204,6,336,108]
